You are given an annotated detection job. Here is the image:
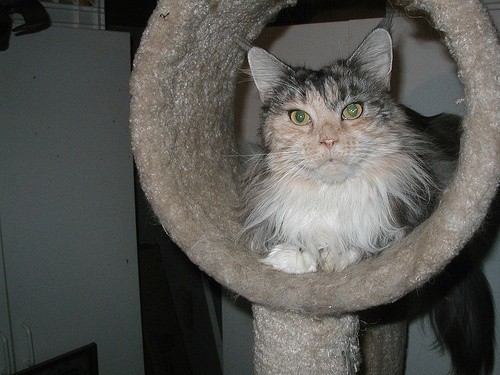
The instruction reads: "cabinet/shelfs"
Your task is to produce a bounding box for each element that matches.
[0,22,145,375]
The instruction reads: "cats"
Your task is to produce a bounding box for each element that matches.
[247,29,497,375]
